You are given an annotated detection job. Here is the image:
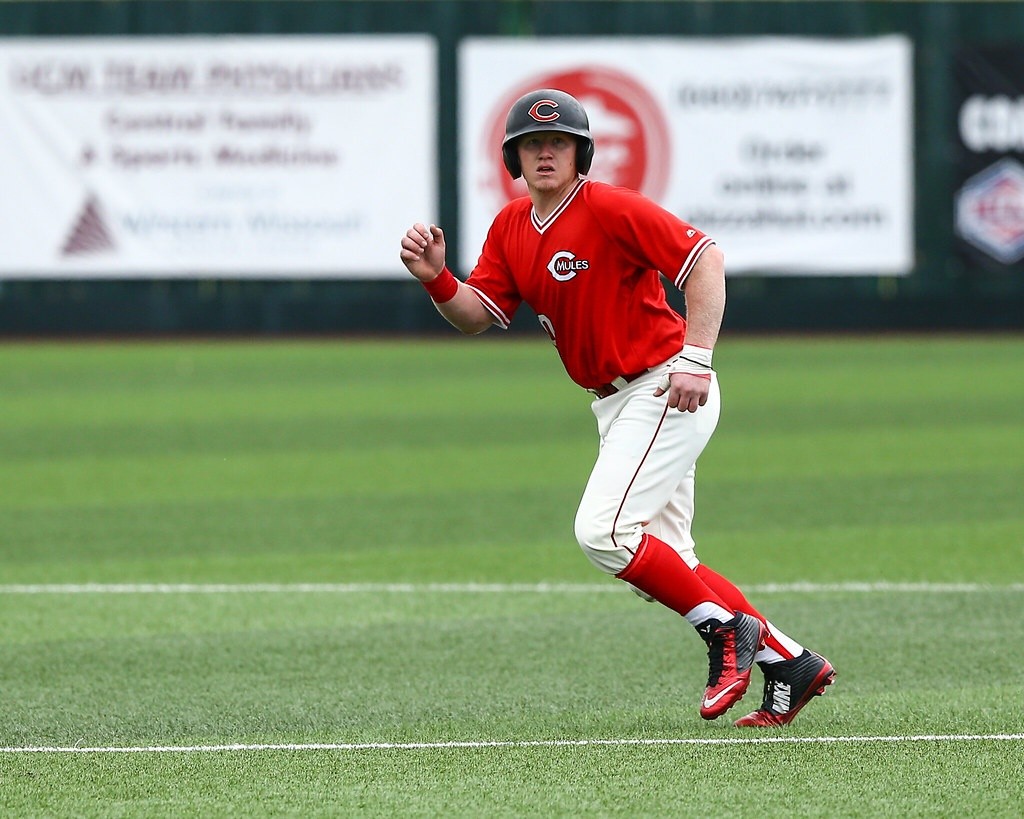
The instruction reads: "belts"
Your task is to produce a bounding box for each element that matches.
[595,366,649,398]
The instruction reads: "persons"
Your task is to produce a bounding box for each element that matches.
[400,89,837,730]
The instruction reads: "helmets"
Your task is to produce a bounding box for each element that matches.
[501,87,595,182]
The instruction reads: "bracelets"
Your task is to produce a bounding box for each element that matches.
[679,356,712,370]
[663,343,713,381]
[420,265,458,303]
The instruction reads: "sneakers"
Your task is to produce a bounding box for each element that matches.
[694,611,772,720]
[734,648,838,729]
